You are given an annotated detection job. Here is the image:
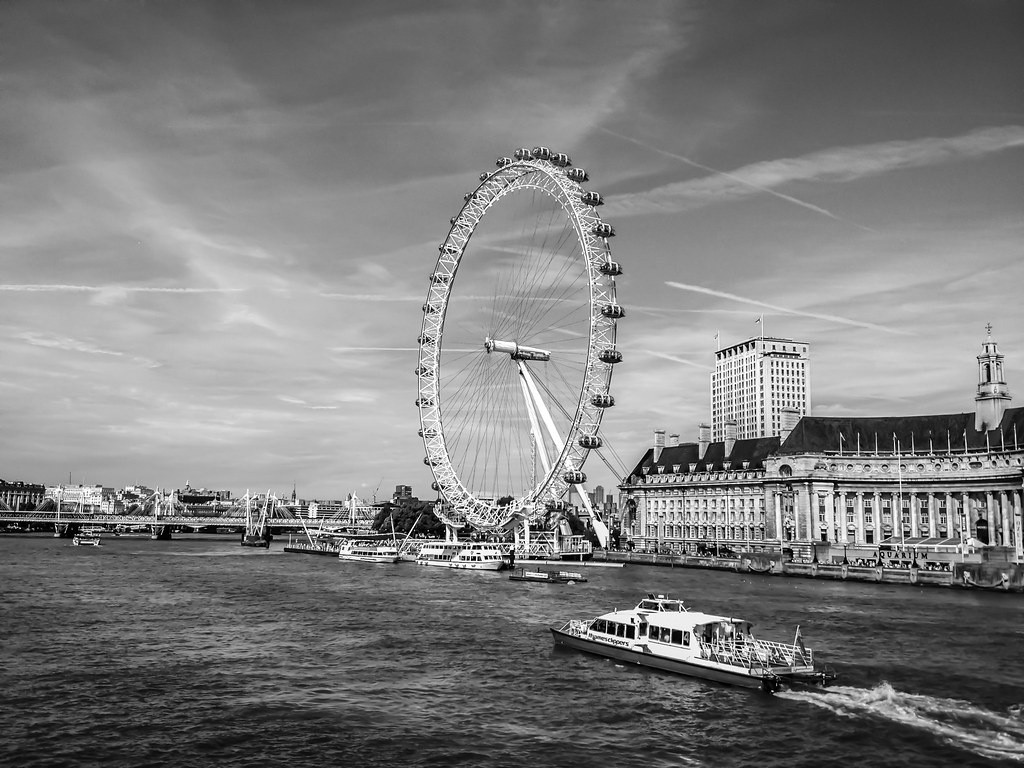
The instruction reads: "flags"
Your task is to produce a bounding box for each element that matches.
[755,317,760,324]
[714,332,718,340]
[963,431,965,436]
[841,432,845,441]
[984,430,987,435]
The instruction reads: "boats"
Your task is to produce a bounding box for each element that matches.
[414,541,515,570]
[548,591,840,697]
[72,530,102,546]
[337,539,402,564]
[448,549,504,571]
